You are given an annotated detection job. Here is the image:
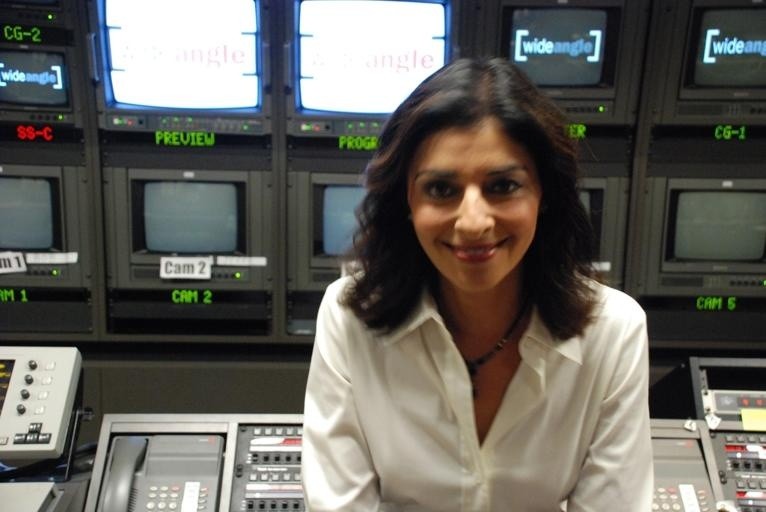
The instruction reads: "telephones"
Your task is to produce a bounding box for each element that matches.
[97,435,224,512]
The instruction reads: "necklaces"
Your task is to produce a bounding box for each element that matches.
[431,284,531,393]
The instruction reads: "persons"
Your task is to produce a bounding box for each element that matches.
[298,54,657,510]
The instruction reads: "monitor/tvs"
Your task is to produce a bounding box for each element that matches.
[293,170,369,292]
[287,0,463,139]
[677,0,766,103]
[574,176,627,287]
[93,0,274,138]
[0,163,68,264]
[126,166,270,268]
[0,44,74,115]
[498,0,624,102]
[661,169,765,273]
